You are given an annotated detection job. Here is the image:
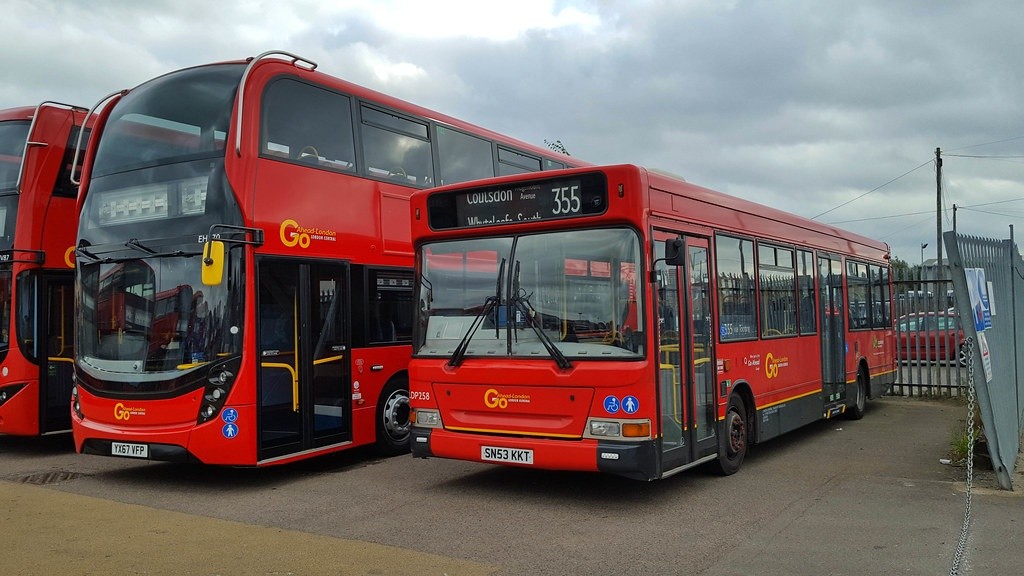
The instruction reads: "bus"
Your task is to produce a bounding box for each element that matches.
[411,165,896,484]
[67,50,666,470]
[0,100,414,435]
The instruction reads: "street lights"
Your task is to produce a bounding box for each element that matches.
[919,242,929,293]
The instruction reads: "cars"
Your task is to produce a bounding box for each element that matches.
[892,311,966,368]
